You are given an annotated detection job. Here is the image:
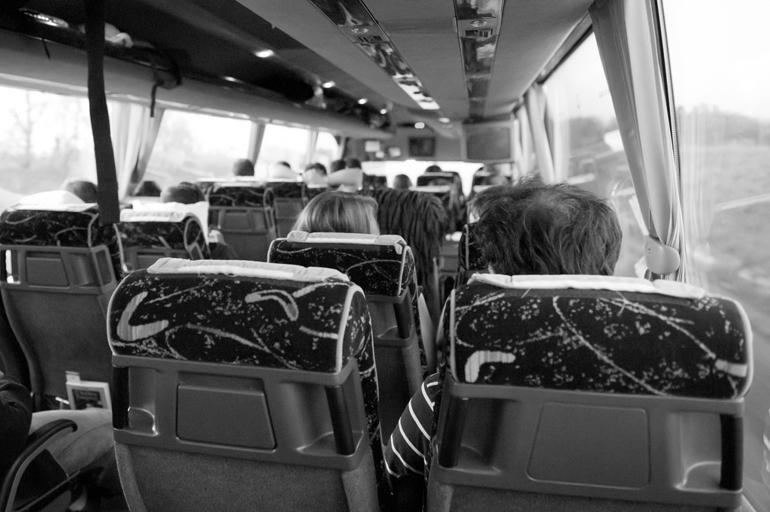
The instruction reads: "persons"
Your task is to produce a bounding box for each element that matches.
[63,158,511,356]
[1,368,128,512]
[383,176,624,495]
